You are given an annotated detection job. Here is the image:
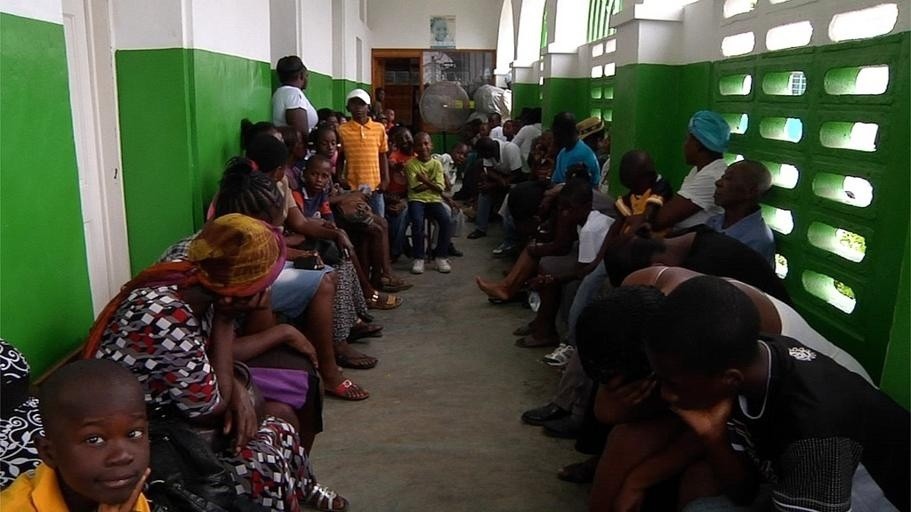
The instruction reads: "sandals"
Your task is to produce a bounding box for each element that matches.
[298,484,350,512]
[324,273,414,401]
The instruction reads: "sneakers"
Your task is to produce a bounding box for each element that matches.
[467,229,486,239]
[433,258,451,273]
[412,258,424,274]
[491,239,522,257]
[514,317,580,440]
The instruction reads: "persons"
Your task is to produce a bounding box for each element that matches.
[499,72,513,129]
[454,106,911,512]
[0,357,162,512]
[431,17,456,50]
[85,53,474,512]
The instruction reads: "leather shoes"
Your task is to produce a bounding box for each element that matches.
[558,454,602,482]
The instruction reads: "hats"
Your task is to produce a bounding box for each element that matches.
[575,115,606,140]
[345,89,373,107]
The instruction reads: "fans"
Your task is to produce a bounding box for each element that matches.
[419,78,472,154]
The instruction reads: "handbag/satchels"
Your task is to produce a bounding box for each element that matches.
[143,423,252,511]
